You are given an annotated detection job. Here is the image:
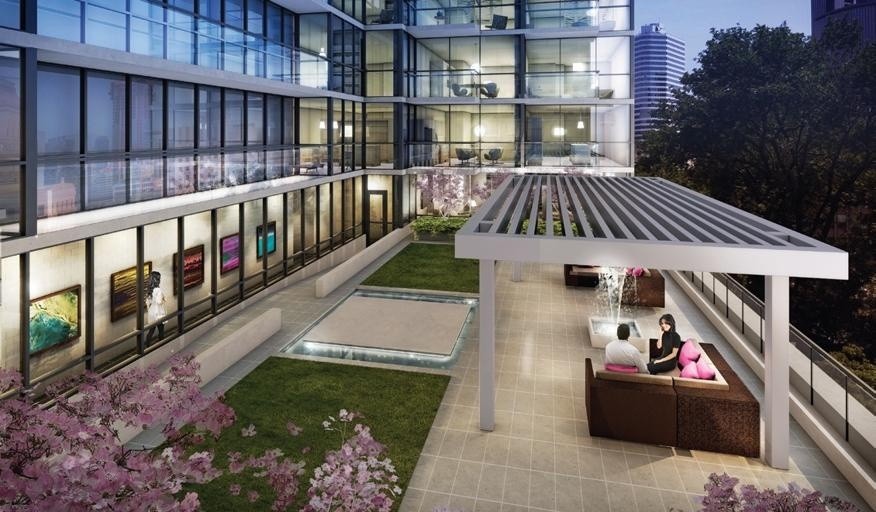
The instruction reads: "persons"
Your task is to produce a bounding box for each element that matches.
[603,313,680,374]
[146,271,167,347]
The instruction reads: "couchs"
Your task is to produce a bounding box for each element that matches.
[585,337,760,459]
[564,264,666,308]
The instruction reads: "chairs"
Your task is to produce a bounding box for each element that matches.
[485,14,508,30]
[452,83,500,98]
[455,147,503,167]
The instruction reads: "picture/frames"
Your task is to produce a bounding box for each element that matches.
[30,220,277,358]
